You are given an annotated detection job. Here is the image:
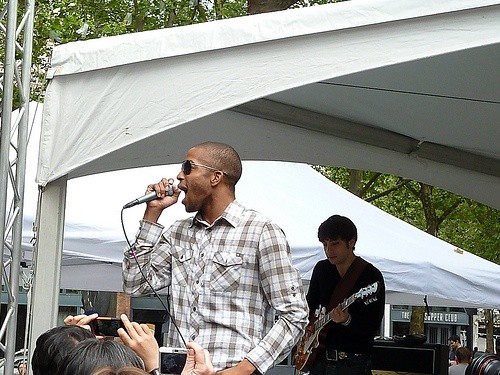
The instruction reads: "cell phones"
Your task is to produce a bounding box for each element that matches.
[89,317,129,337]
[159,346,189,375]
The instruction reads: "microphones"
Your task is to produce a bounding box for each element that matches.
[124,185,174,209]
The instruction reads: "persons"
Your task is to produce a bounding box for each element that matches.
[445,334,478,375]
[294,214,387,375]
[119,139,311,375]
[17,314,216,374]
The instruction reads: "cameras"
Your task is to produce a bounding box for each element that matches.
[464,353,500,375]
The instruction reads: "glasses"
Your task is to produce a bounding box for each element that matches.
[181,159,229,178]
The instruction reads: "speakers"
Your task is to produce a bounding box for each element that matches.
[370,339,449,375]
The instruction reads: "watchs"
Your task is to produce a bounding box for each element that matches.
[147,366,164,375]
[341,314,351,327]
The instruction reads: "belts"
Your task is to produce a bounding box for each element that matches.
[316,349,371,361]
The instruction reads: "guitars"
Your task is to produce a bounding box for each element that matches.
[294,282,378,373]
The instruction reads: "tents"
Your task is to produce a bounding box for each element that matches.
[26,2,500,373]
[1,101,500,309]
